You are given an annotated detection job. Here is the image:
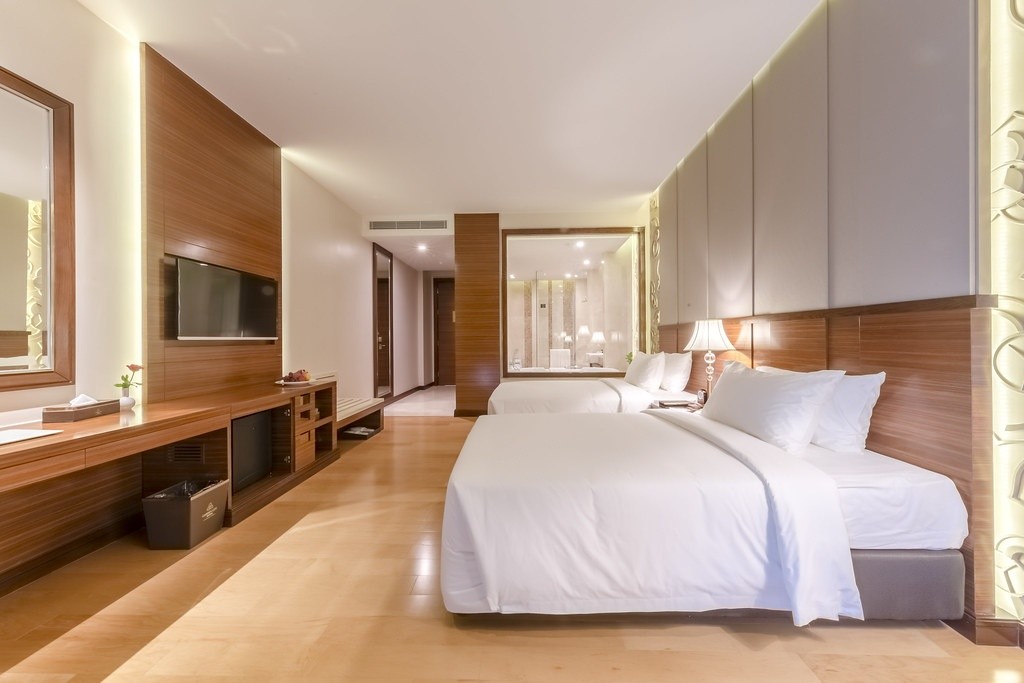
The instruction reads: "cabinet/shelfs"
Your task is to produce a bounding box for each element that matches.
[0,379,384,581]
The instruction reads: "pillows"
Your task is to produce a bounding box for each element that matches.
[624,351,666,394]
[755,366,887,458]
[660,351,692,394]
[700,361,846,456]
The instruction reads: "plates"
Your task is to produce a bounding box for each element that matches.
[275,378,317,386]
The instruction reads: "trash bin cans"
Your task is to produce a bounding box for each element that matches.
[141,480,231,550]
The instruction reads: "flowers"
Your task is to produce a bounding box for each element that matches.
[114,364,144,389]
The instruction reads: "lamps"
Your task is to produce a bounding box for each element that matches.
[590,331,607,353]
[683,319,736,403]
[734,319,781,367]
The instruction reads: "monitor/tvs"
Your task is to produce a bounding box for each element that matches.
[175,258,278,341]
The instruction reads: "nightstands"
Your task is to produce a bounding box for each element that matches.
[650,400,701,409]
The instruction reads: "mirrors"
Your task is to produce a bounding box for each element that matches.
[0,66,79,392]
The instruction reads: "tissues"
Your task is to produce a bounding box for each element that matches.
[41,394,120,423]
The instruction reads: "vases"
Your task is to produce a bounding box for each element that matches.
[120,388,135,410]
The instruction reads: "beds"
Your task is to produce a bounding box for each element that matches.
[488,350,700,416]
[438,360,970,630]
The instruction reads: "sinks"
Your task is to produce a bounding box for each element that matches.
[586,353,604,363]
